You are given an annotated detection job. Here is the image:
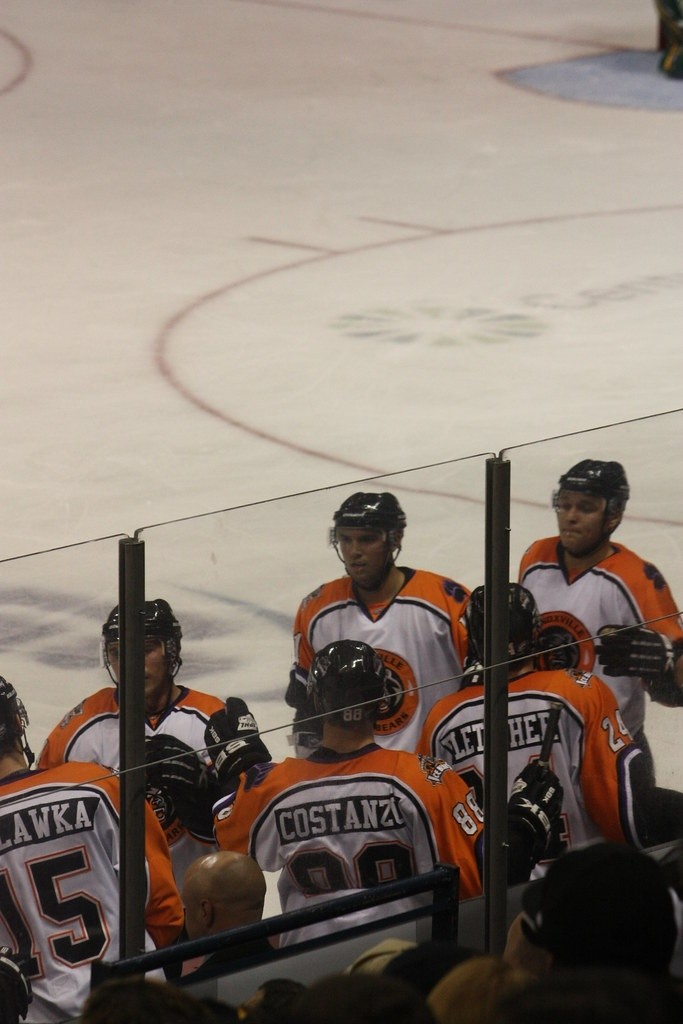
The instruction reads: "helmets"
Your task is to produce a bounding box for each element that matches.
[559,459,629,517]
[465,583,538,665]
[0,675,18,746]
[102,598,181,671]
[307,639,385,720]
[332,492,405,550]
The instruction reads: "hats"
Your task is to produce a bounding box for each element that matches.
[521,840,678,972]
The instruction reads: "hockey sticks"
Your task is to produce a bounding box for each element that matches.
[530,704,566,775]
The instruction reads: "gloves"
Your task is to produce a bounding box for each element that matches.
[507,761,563,865]
[0,946,33,1024]
[594,624,674,678]
[203,698,271,779]
[147,734,214,837]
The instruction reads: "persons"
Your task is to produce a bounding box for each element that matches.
[177,851,320,987]
[37,599,226,895]
[0,675,185,1023]
[415,583,662,880]
[285,492,473,753]
[517,459,683,787]
[78,840,683,1024]
[204,639,484,947]
